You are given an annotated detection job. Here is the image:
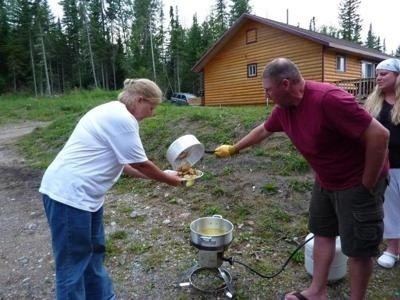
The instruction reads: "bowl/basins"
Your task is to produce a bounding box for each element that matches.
[162,169,204,187]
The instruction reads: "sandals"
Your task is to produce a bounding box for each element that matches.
[377,251,399,269]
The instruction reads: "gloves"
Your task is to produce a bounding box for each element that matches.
[214,144,238,157]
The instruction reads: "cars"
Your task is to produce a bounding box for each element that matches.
[170,93,197,104]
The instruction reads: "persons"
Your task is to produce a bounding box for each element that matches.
[214,57,391,300]
[364,58,400,268]
[39,78,183,300]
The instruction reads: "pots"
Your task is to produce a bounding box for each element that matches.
[189,214,234,248]
[165,134,239,172]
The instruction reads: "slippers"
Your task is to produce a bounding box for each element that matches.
[281,288,310,300]
